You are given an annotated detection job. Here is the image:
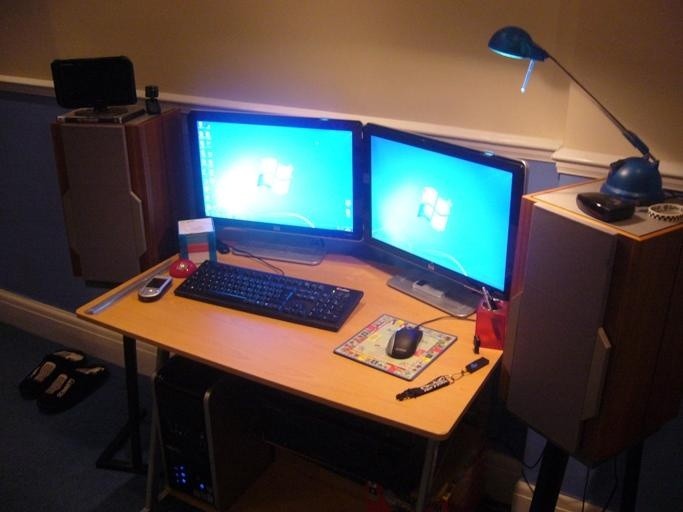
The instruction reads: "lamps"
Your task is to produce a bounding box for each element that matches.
[488,25,667,206]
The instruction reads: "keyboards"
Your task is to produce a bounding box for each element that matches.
[174,259,364,332]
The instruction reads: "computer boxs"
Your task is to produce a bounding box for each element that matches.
[150,353,276,512]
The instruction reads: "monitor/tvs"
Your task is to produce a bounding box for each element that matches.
[362,123,530,318]
[180,105,363,266]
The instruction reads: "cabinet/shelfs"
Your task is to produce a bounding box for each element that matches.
[48,105,183,289]
[501,175,682,512]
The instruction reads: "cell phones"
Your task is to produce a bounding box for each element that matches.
[138,275,173,302]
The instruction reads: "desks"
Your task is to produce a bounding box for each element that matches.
[75,231,504,512]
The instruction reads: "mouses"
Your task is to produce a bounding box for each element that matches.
[386,325,423,359]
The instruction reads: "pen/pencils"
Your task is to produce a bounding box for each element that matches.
[482,286,499,340]
[488,294,497,310]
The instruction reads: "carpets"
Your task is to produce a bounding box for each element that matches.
[0,319,201,511]
